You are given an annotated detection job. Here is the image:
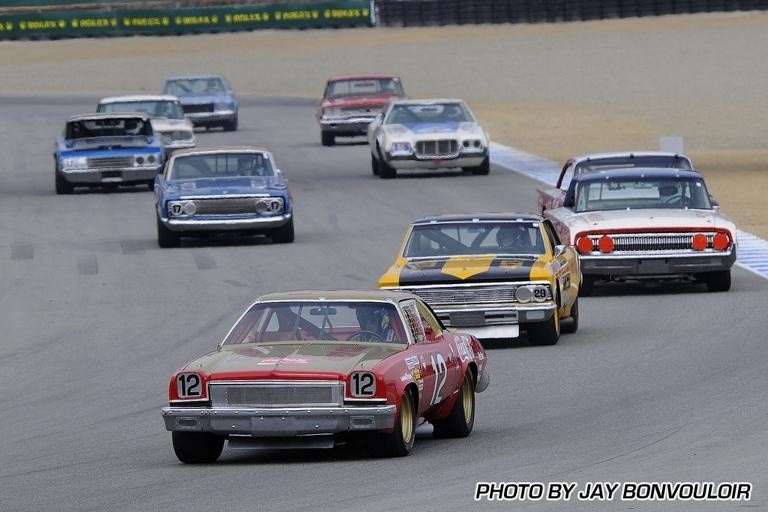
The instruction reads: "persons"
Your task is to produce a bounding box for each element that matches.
[354,311,398,342]
